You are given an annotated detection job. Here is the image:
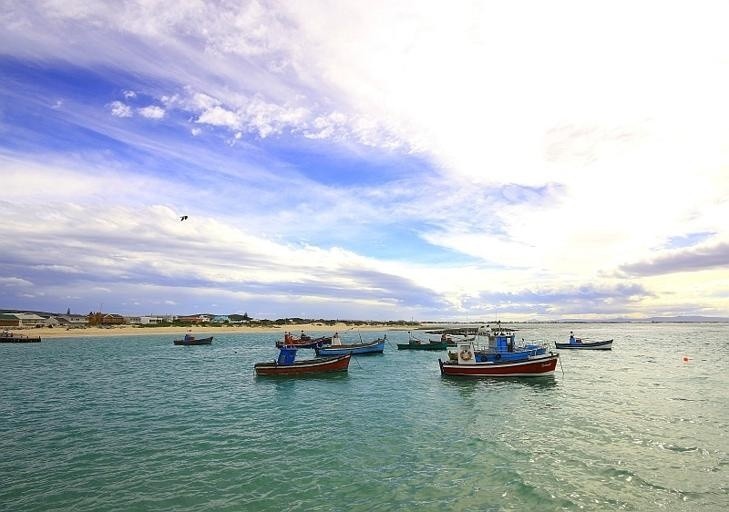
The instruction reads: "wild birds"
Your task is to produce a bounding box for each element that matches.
[180,215,188,221]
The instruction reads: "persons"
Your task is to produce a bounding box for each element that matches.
[333,333,338,338]
[301,330,304,336]
[570,331,574,339]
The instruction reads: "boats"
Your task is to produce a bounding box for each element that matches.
[397,327,613,377]
[255,334,387,375]
[174,334,213,345]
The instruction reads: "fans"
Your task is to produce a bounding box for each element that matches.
[458,344,476,365]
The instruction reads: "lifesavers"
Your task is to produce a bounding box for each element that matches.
[460,350,472,360]
